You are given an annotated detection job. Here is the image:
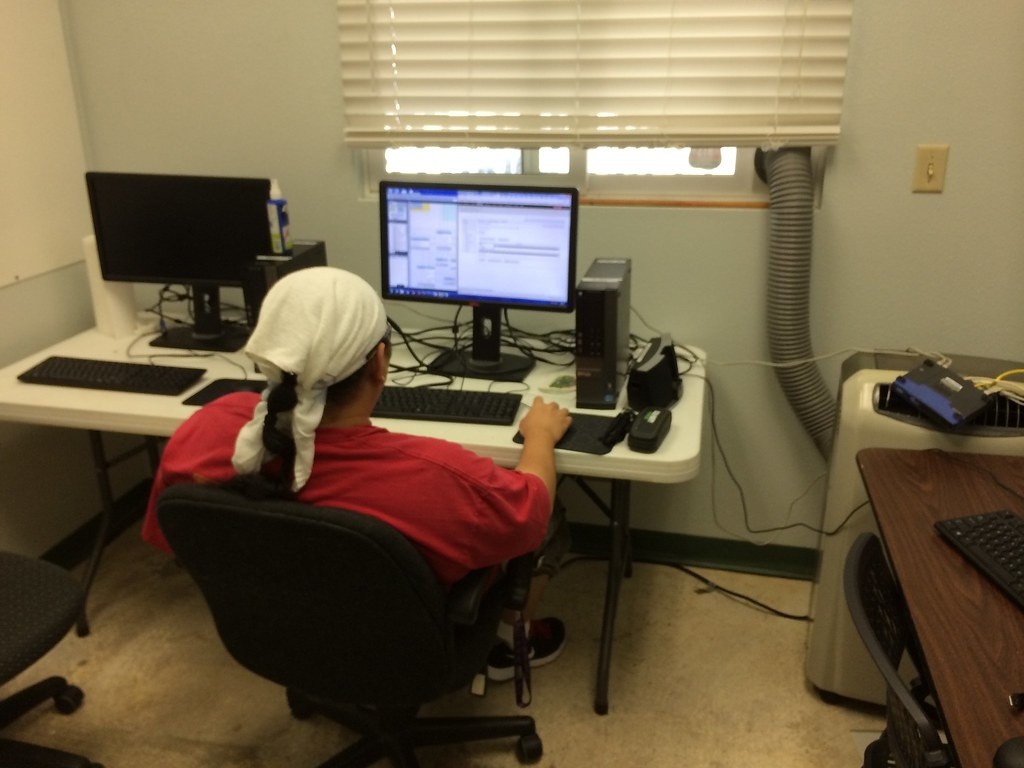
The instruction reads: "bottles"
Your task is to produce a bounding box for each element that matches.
[266,179,292,255]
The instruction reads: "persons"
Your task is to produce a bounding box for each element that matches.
[142,267,573,685]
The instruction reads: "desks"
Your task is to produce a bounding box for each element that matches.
[0,312,710,715]
[857,448,1024,768]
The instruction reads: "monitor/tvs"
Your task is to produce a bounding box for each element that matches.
[86,172,273,353]
[377,179,580,382]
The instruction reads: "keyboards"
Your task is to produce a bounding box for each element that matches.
[15,356,207,396]
[368,385,524,425]
[935,509,1024,613]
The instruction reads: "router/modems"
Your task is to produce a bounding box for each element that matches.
[891,358,993,433]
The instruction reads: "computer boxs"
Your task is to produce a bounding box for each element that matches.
[256,238,329,299]
[575,258,632,410]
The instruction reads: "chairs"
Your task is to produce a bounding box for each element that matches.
[0,550,106,768]
[843,532,955,768]
[155,484,545,768]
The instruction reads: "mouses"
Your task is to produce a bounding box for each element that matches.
[238,381,257,392]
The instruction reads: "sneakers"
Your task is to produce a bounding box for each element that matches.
[485,616,567,681]
